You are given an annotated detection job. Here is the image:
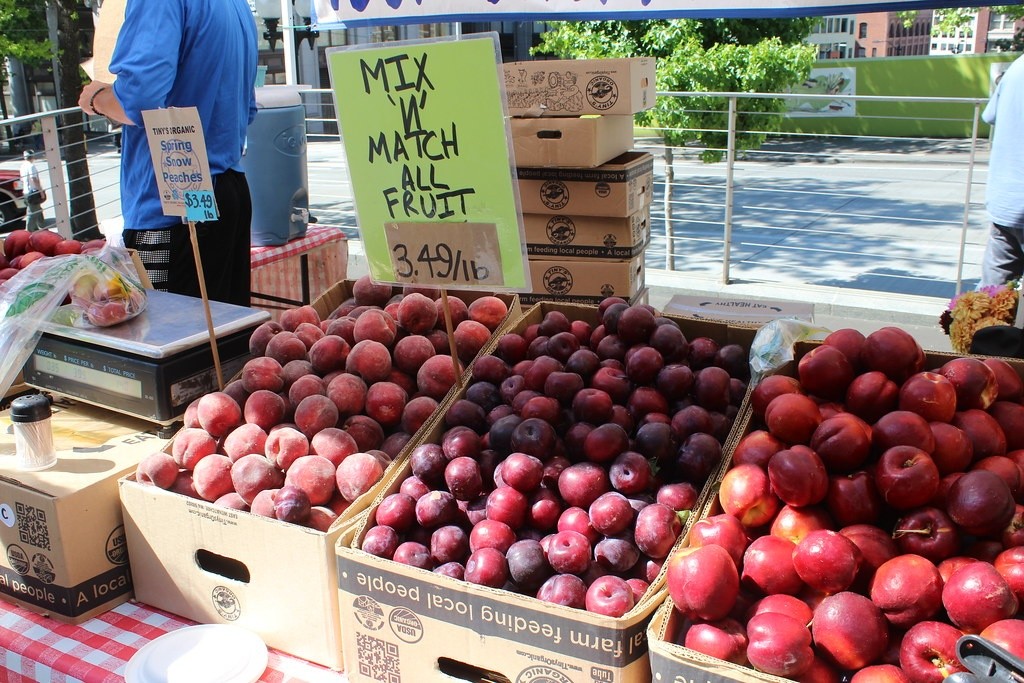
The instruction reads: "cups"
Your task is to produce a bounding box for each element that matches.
[255,66,267,87]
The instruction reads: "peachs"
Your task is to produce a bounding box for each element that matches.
[0,229,142,325]
[137,281,508,532]
[665,328,1024,683]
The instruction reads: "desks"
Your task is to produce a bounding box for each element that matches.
[251,224,348,311]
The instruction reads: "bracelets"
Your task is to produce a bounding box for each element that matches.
[90,86,104,117]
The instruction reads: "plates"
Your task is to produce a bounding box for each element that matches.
[123,622,269,683]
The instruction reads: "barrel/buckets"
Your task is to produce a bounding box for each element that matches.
[240,85,310,247]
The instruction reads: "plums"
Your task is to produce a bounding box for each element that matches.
[359,296,746,616]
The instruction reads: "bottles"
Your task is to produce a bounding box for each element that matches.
[12,395,58,473]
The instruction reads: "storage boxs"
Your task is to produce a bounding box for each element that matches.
[501,57,656,310]
[0,249,1024,683]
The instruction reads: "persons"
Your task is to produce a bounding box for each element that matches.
[977,53,1024,290]
[20,150,48,232]
[78,0,258,308]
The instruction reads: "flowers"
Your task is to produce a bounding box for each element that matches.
[938,279,1019,353]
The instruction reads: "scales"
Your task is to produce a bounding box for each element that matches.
[21,286,276,440]
